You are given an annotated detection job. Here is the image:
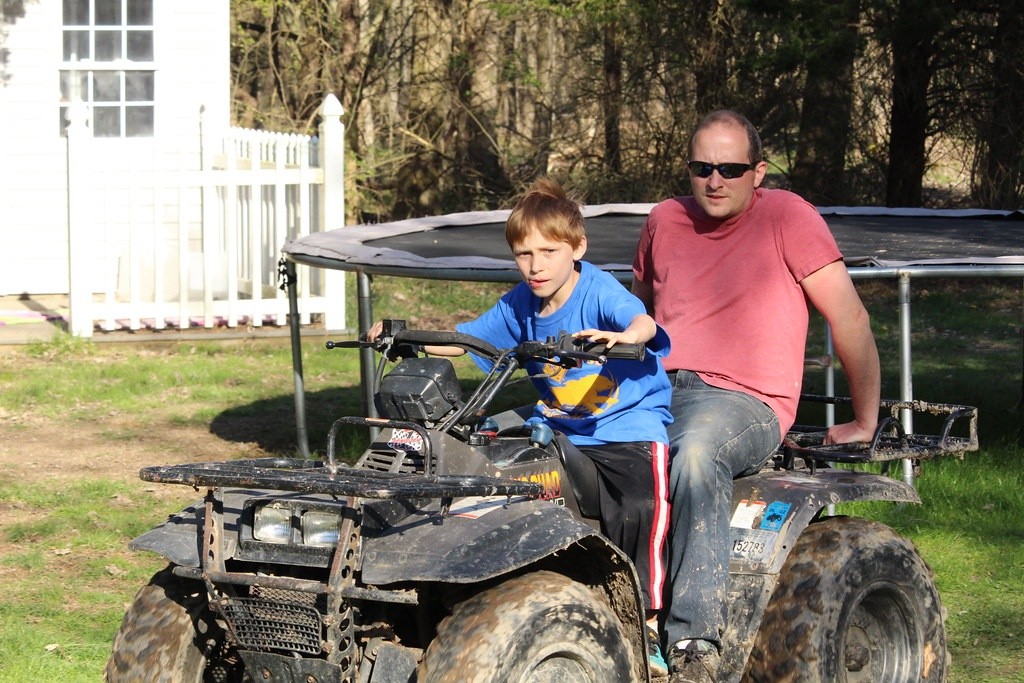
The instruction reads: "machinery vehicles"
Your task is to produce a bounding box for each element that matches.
[105,208,1024,683]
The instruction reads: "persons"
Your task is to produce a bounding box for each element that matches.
[630,110,881,683]
[366,178,672,676]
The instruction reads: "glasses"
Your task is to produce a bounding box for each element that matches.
[686,160,756,179]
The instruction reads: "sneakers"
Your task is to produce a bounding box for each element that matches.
[668,639,719,683]
[646,626,669,677]
